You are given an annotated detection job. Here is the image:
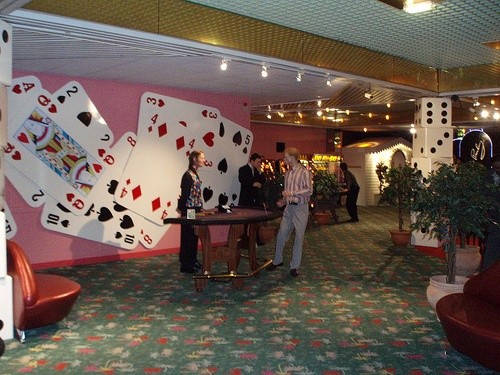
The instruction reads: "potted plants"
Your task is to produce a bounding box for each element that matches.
[312,170,341,226]
[375,161,425,247]
[257,172,284,242]
[409,158,500,323]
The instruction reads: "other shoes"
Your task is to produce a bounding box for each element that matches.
[192,260,203,268]
[265,262,284,271]
[180,262,198,274]
[347,217,358,222]
[257,240,265,246]
[290,269,299,276]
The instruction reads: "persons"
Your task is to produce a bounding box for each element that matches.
[340,162,360,222]
[177,151,205,274]
[477,156,500,271]
[238,153,266,208]
[266,148,313,276]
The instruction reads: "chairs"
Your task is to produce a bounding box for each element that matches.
[436,260,500,371]
[7,239,81,343]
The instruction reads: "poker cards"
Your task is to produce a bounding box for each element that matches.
[1,73,255,251]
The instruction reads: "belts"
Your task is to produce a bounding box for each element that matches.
[289,201,298,205]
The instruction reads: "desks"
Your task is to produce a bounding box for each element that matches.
[163,209,275,290]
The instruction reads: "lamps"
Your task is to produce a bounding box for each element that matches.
[261,65,268,77]
[220,58,228,71]
[296,73,302,82]
